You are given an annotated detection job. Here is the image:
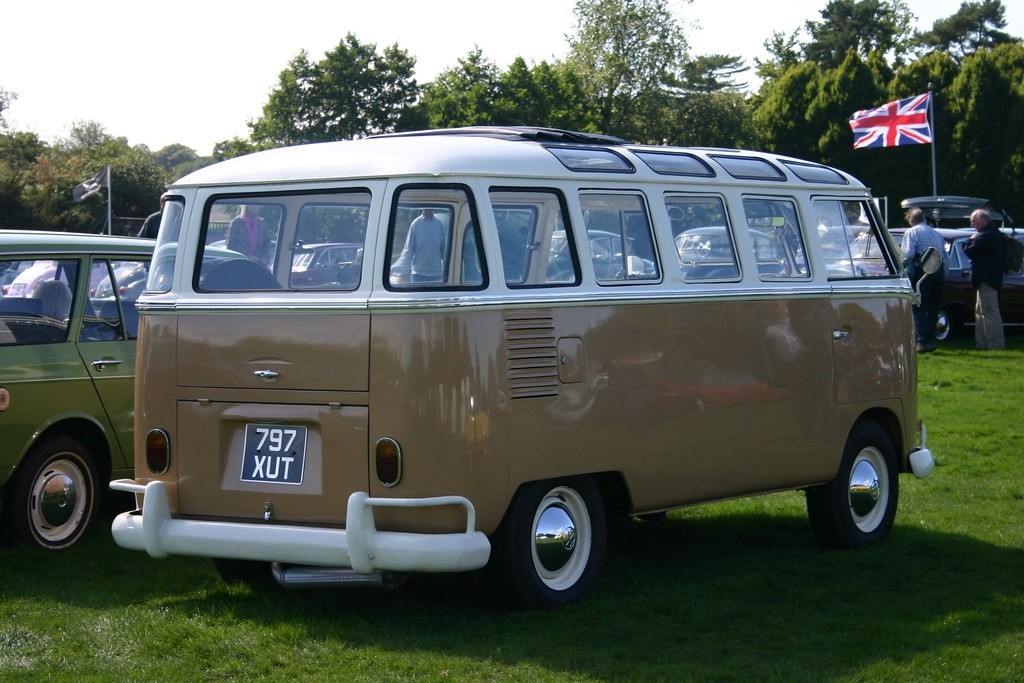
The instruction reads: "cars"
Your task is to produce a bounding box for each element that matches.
[0,195,1024,570]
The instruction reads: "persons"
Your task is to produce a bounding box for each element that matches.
[33,279,73,322]
[392,210,447,286]
[135,190,168,238]
[225,205,275,290]
[961,209,1008,353]
[901,208,950,352]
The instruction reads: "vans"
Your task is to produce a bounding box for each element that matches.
[110,127,934,602]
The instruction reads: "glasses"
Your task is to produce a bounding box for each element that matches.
[971,220,978,225]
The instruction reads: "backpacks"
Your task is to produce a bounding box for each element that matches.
[1000,232,1024,274]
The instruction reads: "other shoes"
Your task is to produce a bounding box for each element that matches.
[915,342,937,353]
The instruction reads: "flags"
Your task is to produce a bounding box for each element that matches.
[849,92,933,149]
[72,167,109,204]
